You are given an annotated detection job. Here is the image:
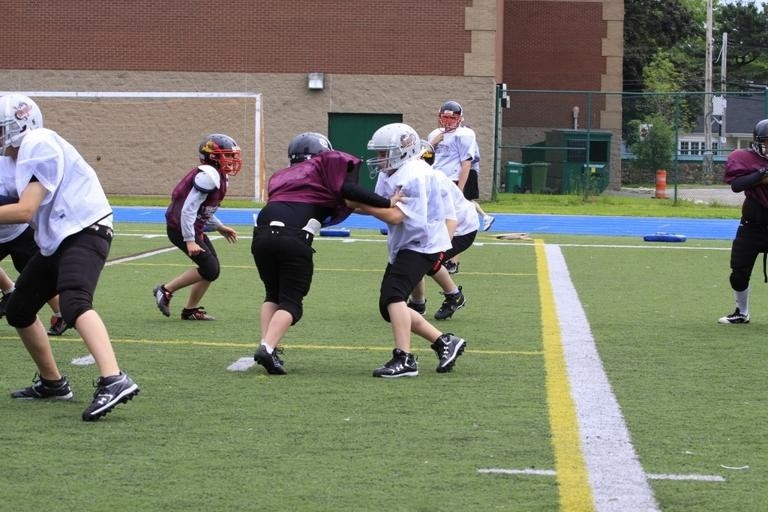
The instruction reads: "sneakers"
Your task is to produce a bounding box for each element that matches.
[83,370,140,419]
[47,314,69,335]
[252,343,289,375]
[718,307,750,324]
[152,284,173,317]
[435,285,467,319]
[11,372,73,401]
[406,298,427,316]
[0,290,13,319]
[373,348,419,377]
[482,215,495,231]
[181,307,214,320]
[444,261,459,274]
[430,332,466,372]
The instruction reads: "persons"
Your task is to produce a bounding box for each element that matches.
[1,91,140,421]
[153,134,241,322]
[0,155,74,338]
[352,123,466,378]
[717,119,768,326]
[464,143,495,232]
[250,130,410,374]
[429,100,476,210]
[397,135,481,320]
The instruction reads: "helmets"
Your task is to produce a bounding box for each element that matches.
[366,123,426,180]
[421,139,435,166]
[198,134,243,176]
[751,119,768,160]
[436,100,463,133]
[288,132,334,161]
[0,92,44,157]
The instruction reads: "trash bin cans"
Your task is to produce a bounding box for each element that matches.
[529,162,552,194]
[504,164,527,193]
